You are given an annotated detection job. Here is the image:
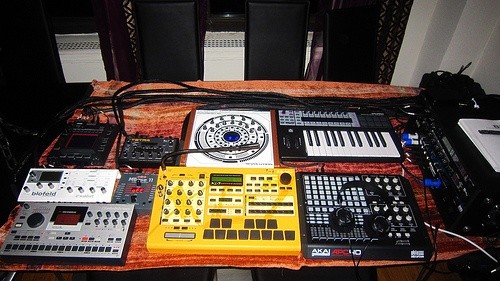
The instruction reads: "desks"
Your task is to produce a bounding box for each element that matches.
[1,78,500,281]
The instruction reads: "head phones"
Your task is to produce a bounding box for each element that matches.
[330,180,393,236]
